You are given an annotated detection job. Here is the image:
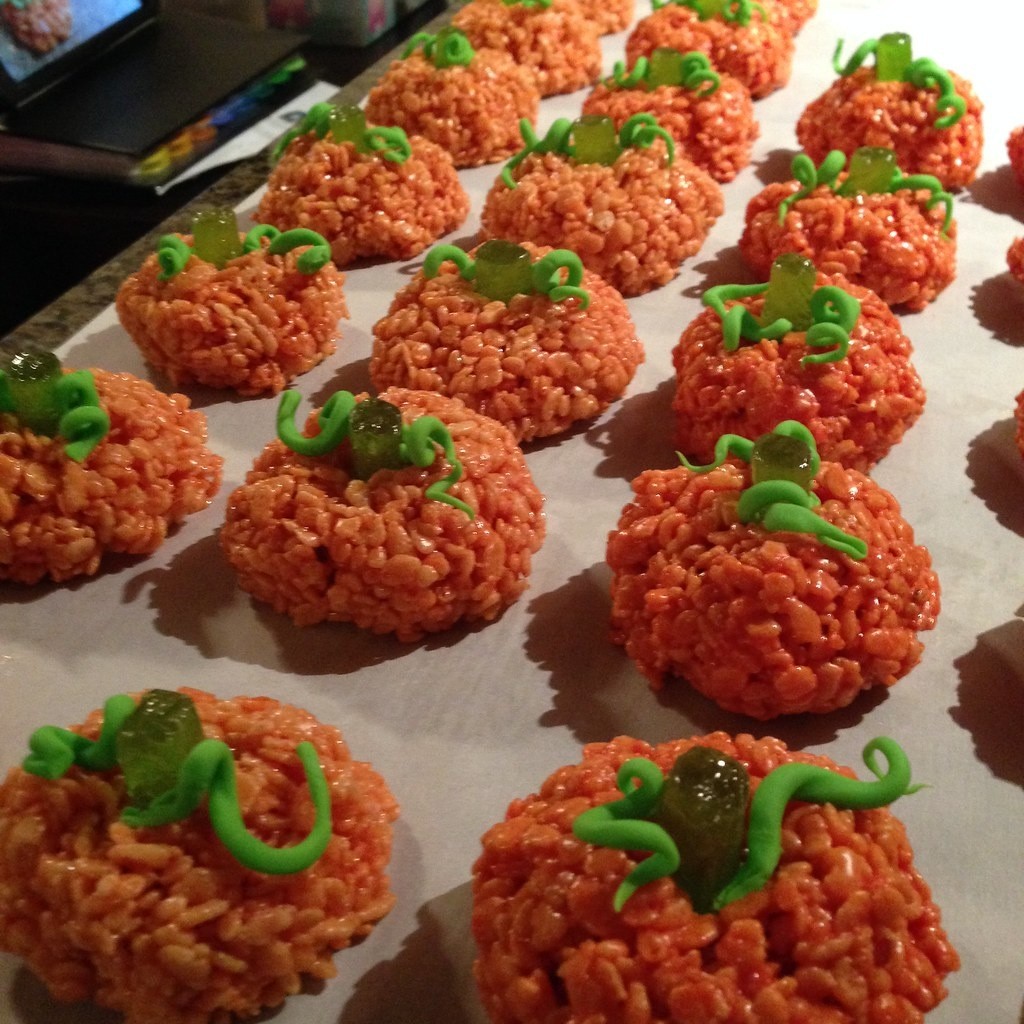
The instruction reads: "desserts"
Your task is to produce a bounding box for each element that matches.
[0,0,1024,1024]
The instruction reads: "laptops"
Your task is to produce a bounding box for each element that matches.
[0,0,314,159]
